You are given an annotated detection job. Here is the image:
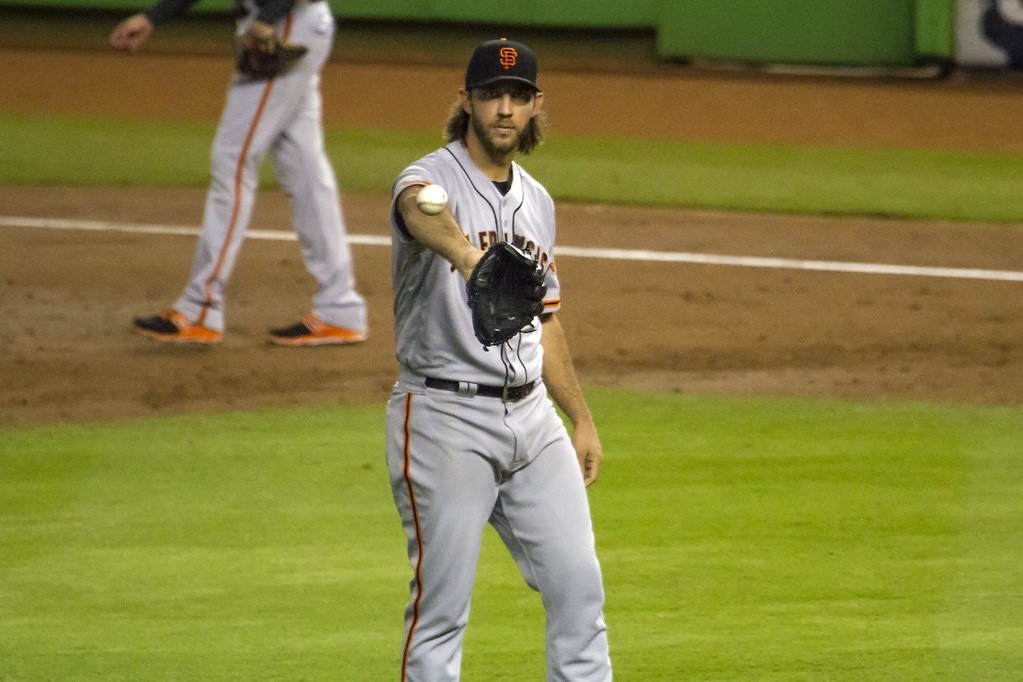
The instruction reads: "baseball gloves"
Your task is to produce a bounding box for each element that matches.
[233,36,309,85]
[464,240,549,353]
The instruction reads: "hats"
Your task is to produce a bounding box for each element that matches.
[466,37,541,93]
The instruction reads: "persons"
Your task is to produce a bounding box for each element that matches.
[109,0,369,345]
[383,35,614,682]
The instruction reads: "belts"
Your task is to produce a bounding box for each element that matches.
[425,377,535,401]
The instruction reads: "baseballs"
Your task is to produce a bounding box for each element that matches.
[415,183,450,217]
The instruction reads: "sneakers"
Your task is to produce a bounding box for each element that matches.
[132,310,224,345]
[269,317,368,347]
